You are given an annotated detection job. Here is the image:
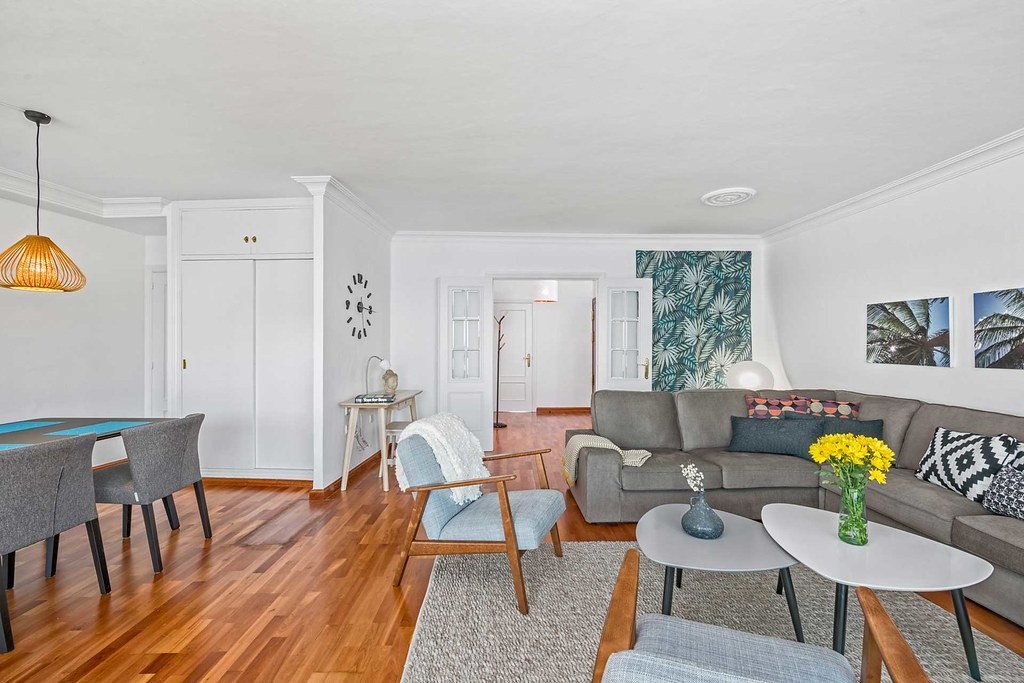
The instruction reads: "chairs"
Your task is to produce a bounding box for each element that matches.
[0,432,111,654]
[93,413,212,572]
[592,549,933,683]
[392,412,566,615]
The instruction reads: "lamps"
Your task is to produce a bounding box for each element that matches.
[0,110,86,293]
[366,355,391,395]
[533,280,558,303]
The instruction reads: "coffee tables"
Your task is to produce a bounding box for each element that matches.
[636,503,804,644]
[760,503,994,683]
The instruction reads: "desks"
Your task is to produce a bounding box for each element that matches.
[339,390,423,492]
[0,418,183,590]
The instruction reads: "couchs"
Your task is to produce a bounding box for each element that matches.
[565,389,1024,627]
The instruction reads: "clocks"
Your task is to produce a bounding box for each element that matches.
[345,273,377,339]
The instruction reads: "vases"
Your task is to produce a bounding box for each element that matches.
[682,492,724,539]
[838,489,868,546]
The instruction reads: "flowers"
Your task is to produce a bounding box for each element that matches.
[679,461,705,492]
[808,432,896,546]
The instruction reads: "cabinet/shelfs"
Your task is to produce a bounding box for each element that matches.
[167,198,314,480]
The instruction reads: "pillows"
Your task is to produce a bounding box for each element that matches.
[724,394,883,462]
[914,427,1024,519]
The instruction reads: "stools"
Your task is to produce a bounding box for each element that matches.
[378,421,412,478]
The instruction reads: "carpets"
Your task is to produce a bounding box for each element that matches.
[399,540,1024,683]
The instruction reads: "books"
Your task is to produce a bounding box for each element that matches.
[355,393,396,403]
